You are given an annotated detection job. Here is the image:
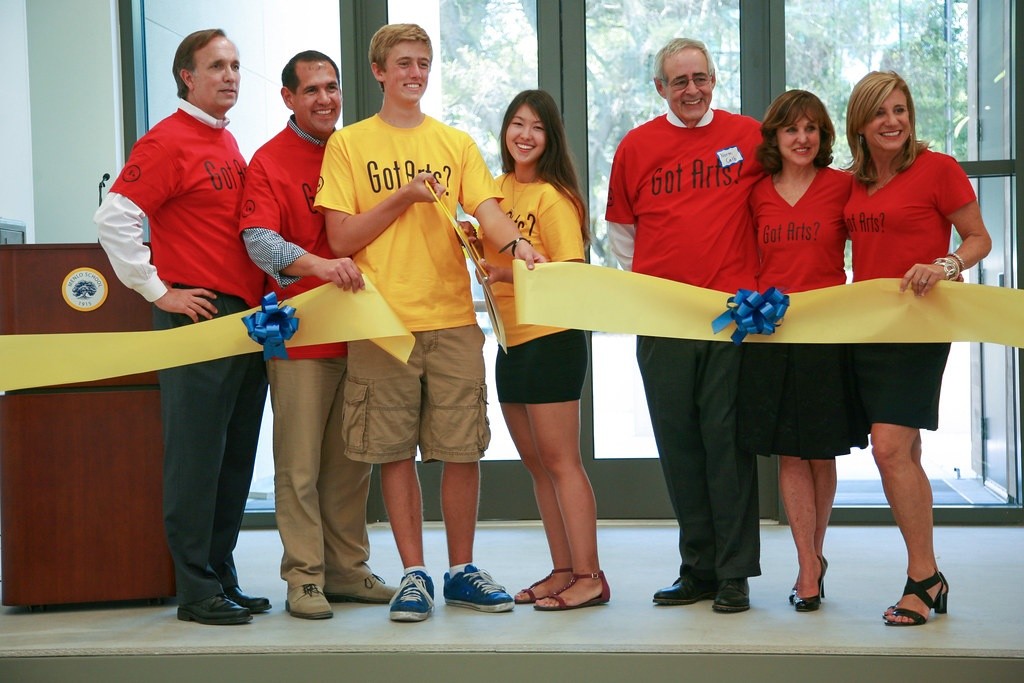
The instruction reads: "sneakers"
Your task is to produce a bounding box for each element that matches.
[286,582,333,619]
[389,570,437,621]
[443,563,515,613]
[325,572,399,601]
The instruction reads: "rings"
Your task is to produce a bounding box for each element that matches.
[920,280,927,284]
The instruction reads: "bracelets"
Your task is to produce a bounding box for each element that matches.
[499,236,531,257]
[947,252,965,272]
[464,228,477,247]
[933,258,960,281]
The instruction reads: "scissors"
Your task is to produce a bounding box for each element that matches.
[425,181,510,352]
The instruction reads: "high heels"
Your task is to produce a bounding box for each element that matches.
[882,570,950,615]
[884,569,943,626]
[794,556,827,614]
[791,556,828,601]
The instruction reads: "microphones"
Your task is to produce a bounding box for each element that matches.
[99,173,110,207]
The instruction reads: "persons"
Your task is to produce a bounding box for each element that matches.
[240,50,400,620]
[313,23,546,621]
[458,89,611,612]
[843,71,992,626]
[750,88,851,611]
[93,28,272,624]
[604,38,765,613]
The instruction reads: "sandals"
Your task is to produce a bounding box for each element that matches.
[533,573,611,611]
[513,568,572,605]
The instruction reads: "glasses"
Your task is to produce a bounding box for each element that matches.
[657,73,709,91]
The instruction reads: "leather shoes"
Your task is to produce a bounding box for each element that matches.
[176,595,252,624]
[712,577,751,610]
[651,566,714,602]
[227,588,273,613]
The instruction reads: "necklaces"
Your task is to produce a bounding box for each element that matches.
[511,177,533,216]
[876,171,893,190]
